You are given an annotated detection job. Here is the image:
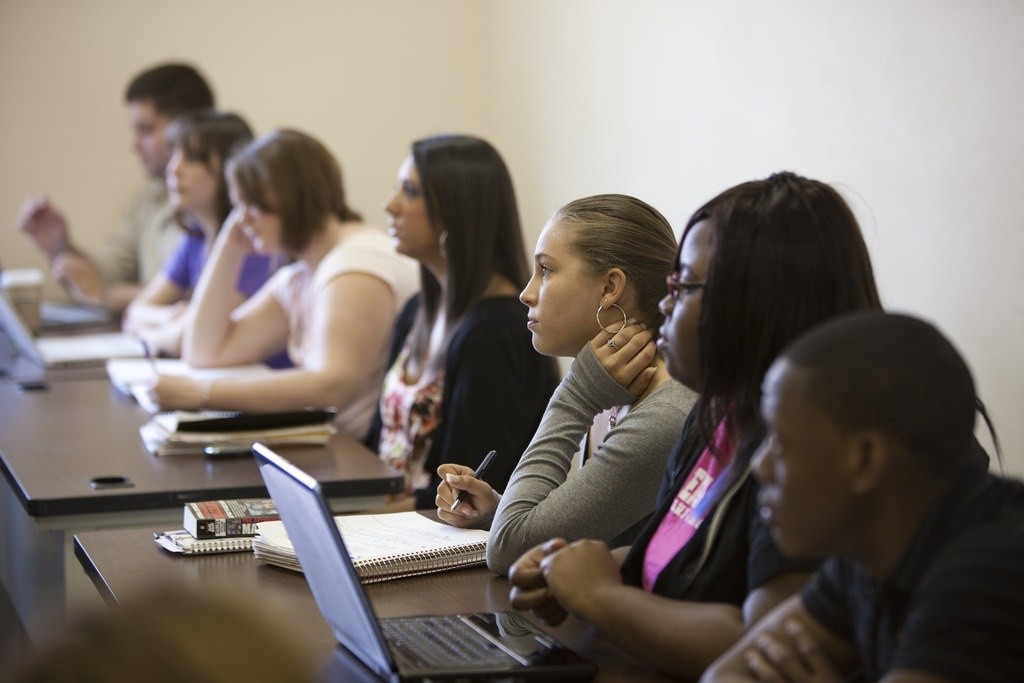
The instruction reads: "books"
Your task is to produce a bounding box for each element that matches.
[251,511,488,586]
[152,498,282,556]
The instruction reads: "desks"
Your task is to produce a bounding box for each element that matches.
[0,313,406,658]
[71,509,694,683]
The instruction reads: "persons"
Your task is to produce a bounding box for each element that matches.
[362,125,886,683]
[19,60,418,443]
[700,312,1023,683]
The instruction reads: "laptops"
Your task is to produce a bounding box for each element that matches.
[0,292,152,369]
[253,440,601,683]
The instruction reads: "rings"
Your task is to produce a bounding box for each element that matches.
[607,338,620,351]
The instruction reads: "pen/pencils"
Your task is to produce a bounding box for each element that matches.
[449,449,496,512]
[138,336,163,379]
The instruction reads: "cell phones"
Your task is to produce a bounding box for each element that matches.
[204,446,253,458]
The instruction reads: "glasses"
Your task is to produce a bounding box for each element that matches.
[664,275,706,299]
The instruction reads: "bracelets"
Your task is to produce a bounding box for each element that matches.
[200,378,216,407]
